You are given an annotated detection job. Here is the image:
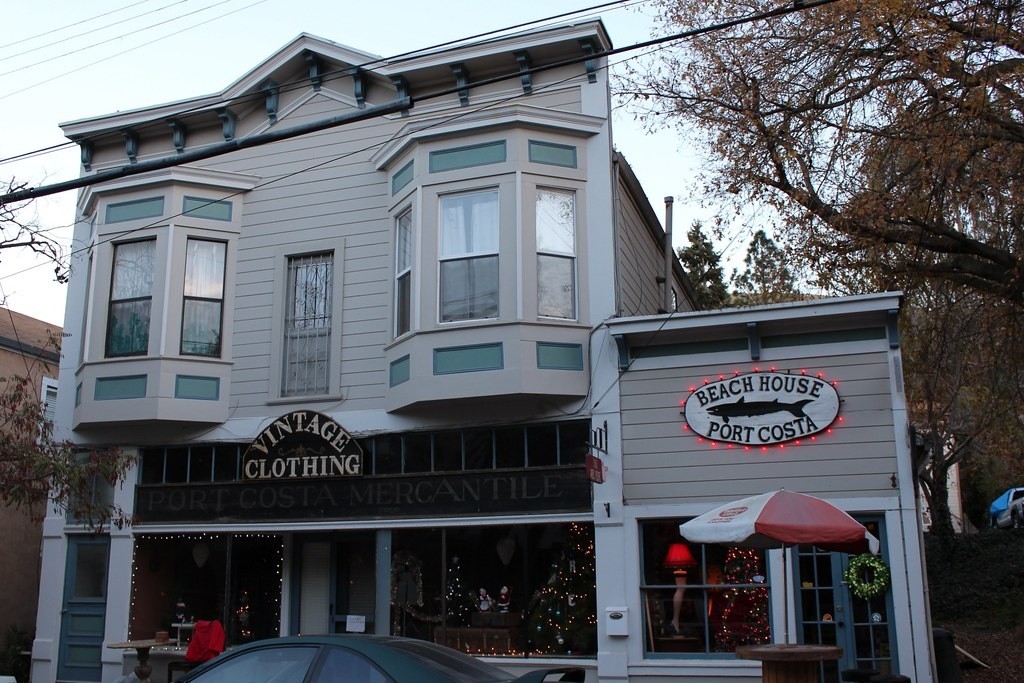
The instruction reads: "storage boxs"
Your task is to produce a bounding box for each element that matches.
[470,611,521,628]
[434,626,523,654]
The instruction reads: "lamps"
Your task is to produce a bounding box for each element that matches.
[664,543,696,576]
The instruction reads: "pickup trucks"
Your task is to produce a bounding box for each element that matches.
[986,486,1024,529]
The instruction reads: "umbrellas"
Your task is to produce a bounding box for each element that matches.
[678,486,879,645]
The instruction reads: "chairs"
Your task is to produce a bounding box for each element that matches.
[167,620,224,683]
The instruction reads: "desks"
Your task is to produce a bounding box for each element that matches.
[736,644,844,683]
[171,623,196,649]
[107,638,179,683]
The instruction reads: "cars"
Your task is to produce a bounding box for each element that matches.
[170,632,586,683]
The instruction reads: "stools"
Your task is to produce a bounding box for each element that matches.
[842,668,911,683]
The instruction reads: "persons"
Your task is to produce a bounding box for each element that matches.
[479,585,510,612]
[129,665,152,683]
